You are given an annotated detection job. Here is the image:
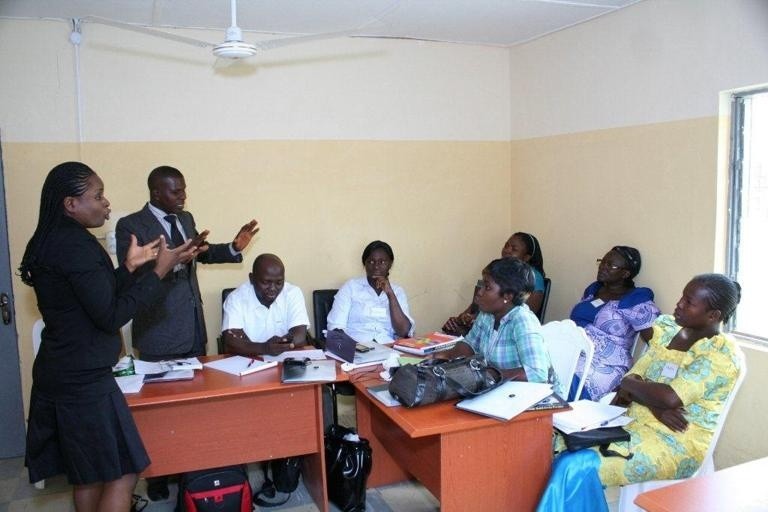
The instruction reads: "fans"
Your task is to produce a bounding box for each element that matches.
[83,0,365,69]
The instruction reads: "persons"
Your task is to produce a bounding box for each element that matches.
[326,240,417,345]
[441,231,547,339]
[115,165,260,502]
[424,258,566,400]
[19,160,198,511]
[564,246,660,403]
[221,253,310,359]
[547,273,741,511]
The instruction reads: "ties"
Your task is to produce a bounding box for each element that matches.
[163,213,193,268]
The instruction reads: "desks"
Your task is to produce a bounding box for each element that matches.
[113,344,346,511]
[553,395,630,454]
[635,454,768,512]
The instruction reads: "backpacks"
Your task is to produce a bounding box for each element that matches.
[175,465,255,512]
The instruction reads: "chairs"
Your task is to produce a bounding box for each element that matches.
[351,343,573,510]
[621,332,745,510]
[222,289,237,312]
[538,279,549,323]
[533,319,592,403]
[314,291,345,342]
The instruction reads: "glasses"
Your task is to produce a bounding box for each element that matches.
[595,258,625,272]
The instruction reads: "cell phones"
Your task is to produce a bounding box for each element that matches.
[355,343,370,352]
[284,356,310,365]
[278,337,294,344]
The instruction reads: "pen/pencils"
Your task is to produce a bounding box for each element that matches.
[177,362,191,366]
[247,359,254,369]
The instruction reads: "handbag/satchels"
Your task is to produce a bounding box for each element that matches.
[253,457,301,507]
[565,426,635,460]
[325,423,372,512]
[388,353,504,409]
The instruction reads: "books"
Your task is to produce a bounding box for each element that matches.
[324,330,356,365]
[280,355,336,383]
[204,353,278,377]
[131,355,205,375]
[394,331,465,356]
[365,383,402,408]
[455,379,569,422]
